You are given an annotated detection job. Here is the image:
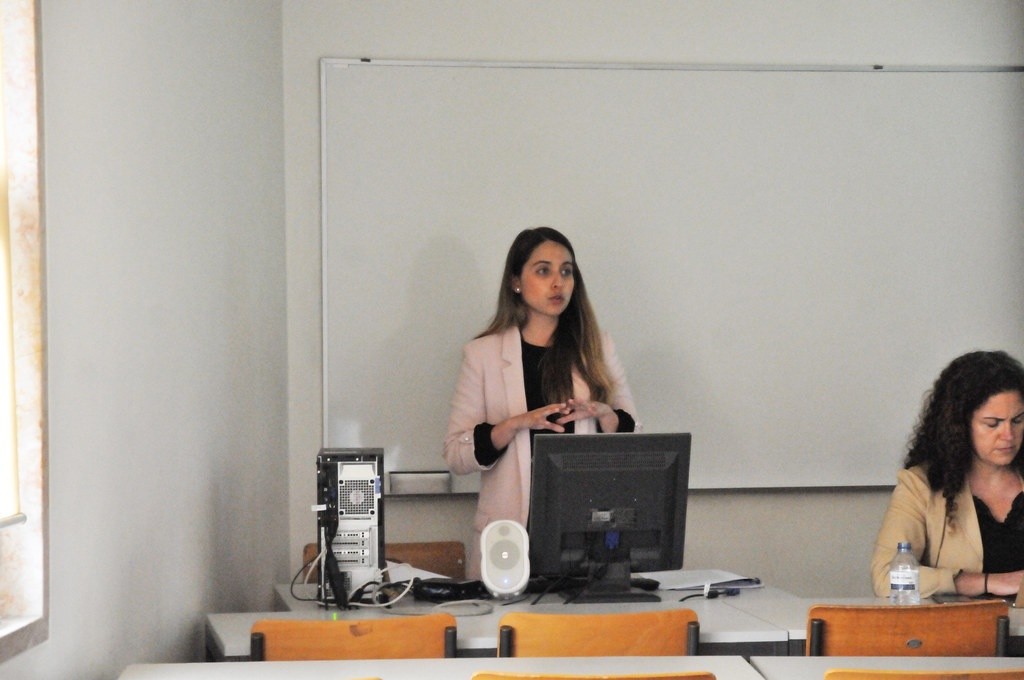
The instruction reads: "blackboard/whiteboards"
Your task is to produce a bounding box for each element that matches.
[320,55,1024,496]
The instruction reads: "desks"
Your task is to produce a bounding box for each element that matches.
[202,569,820,660]
[750,656,1024,680]
[117,654,765,680]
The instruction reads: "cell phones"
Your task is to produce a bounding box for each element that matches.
[931,594,974,603]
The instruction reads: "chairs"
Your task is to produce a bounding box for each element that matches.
[250,613,458,663]
[803,600,1009,658]
[497,609,700,656]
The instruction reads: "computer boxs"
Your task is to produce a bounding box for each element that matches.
[315,447,385,607]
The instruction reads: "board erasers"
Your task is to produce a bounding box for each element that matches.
[389,470,453,496]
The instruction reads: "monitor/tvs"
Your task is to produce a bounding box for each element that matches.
[529,433,692,603]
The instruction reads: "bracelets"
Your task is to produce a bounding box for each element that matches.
[984,573,989,594]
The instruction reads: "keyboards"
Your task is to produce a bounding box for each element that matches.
[525,576,659,595]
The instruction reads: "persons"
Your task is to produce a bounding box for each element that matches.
[442,227,638,580]
[872,350,1024,598]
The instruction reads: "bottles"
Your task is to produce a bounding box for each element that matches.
[890,542,919,604]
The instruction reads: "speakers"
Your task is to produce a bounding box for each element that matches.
[478,519,530,600]
